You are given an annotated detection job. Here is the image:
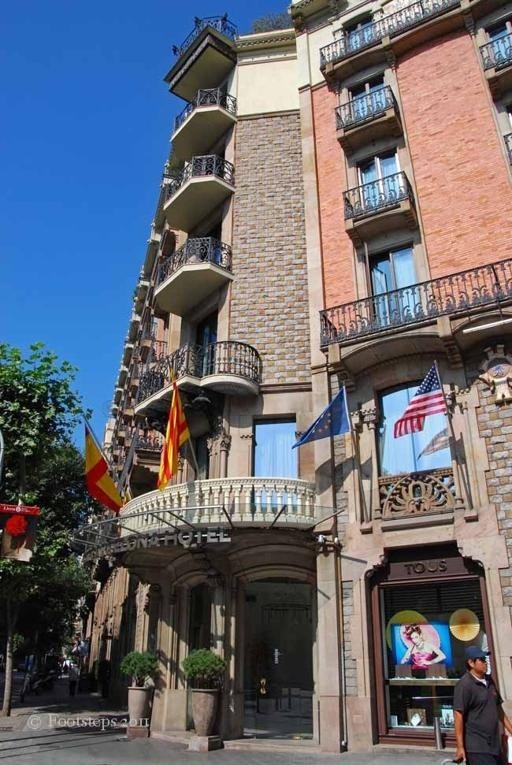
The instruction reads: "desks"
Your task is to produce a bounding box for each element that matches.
[385,675,465,730]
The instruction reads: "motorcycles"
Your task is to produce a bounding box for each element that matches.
[18,664,60,699]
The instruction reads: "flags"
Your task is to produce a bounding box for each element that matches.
[83,423,122,515]
[290,384,353,449]
[394,364,446,439]
[155,381,193,494]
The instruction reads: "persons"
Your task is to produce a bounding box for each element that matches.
[451,645,512,765]
[400,622,448,679]
[62,655,112,697]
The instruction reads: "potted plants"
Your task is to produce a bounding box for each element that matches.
[117,649,165,727]
[180,644,228,738]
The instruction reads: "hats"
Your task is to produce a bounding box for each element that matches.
[464,645,492,662]
